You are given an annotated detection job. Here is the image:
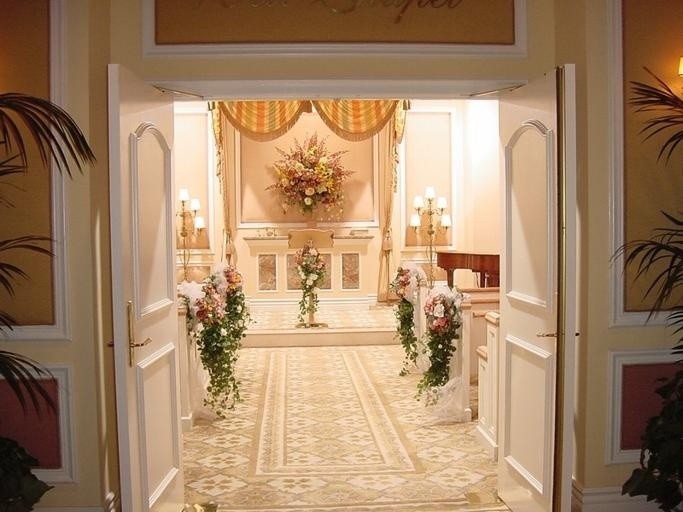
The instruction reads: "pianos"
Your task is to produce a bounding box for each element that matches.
[436,250,500,288]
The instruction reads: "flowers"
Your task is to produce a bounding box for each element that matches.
[389,259,468,409]
[173,267,255,422]
[291,239,331,330]
[260,130,358,222]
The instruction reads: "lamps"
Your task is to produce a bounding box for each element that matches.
[411,186,452,290]
[175,188,206,281]
